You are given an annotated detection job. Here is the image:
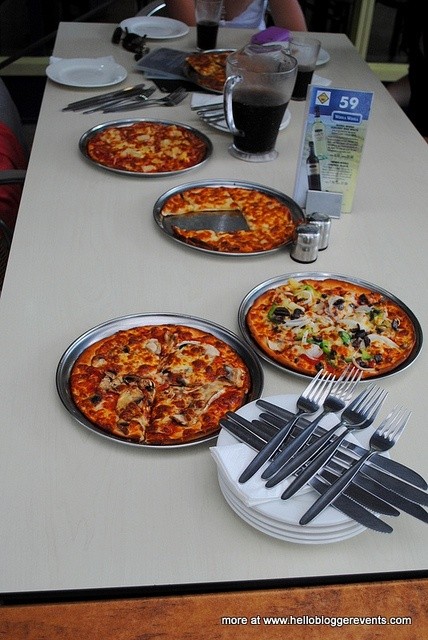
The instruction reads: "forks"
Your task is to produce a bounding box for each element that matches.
[238,368,337,484]
[301,409,413,526]
[101,93,190,114]
[103,86,185,112]
[265,383,386,488]
[261,363,364,480]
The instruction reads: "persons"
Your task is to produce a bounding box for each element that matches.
[386,0,427,140]
[168,0,308,32]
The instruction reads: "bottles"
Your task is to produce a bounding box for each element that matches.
[312,106,328,161]
[306,212,331,252]
[288,223,319,264]
[306,141,322,192]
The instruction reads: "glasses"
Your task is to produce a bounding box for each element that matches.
[113,27,147,53]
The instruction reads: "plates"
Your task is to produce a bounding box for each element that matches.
[46,57,128,88]
[214,393,391,546]
[151,178,306,256]
[78,118,212,178]
[195,93,291,134]
[119,16,190,40]
[237,271,423,382]
[262,41,331,67]
[54,312,263,449]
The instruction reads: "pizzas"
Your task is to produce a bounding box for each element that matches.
[161,186,296,253]
[68,323,252,445]
[87,121,207,174]
[187,49,229,91]
[246,278,416,378]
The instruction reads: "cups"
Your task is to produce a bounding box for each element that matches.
[194,0,224,52]
[222,46,299,164]
[289,36,321,102]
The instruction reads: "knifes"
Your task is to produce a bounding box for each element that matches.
[251,419,428,525]
[62,84,157,114]
[224,410,400,517]
[218,417,394,533]
[255,399,428,492]
[258,412,428,508]
